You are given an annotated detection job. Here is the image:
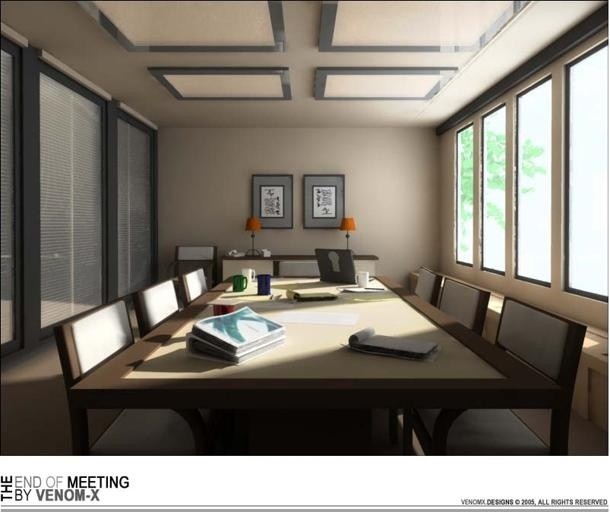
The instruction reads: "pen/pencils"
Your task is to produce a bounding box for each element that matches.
[364,288,384,290]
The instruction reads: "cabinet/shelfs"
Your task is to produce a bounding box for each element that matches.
[218,255,275,279]
[174,258,216,290]
[276,252,381,277]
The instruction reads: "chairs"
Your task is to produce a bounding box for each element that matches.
[437,275,491,339]
[414,266,442,306]
[399,296,590,456]
[57,297,211,455]
[134,275,178,341]
[182,266,207,305]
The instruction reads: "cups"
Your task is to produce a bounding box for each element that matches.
[213,304,235,315]
[241,268,255,283]
[257,274,271,295]
[354,272,370,289]
[233,275,248,292]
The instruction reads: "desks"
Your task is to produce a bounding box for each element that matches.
[67,272,567,456]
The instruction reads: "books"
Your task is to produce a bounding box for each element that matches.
[184,333,287,365]
[286,289,340,303]
[346,326,442,363]
[192,306,283,352]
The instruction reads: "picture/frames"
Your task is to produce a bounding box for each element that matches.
[301,172,346,230]
[249,172,296,231]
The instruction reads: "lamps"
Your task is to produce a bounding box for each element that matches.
[73,0,289,57]
[338,217,354,255]
[146,62,295,103]
[312,65,459,100]
[314,0,533,53]
[243,217,263,257]
